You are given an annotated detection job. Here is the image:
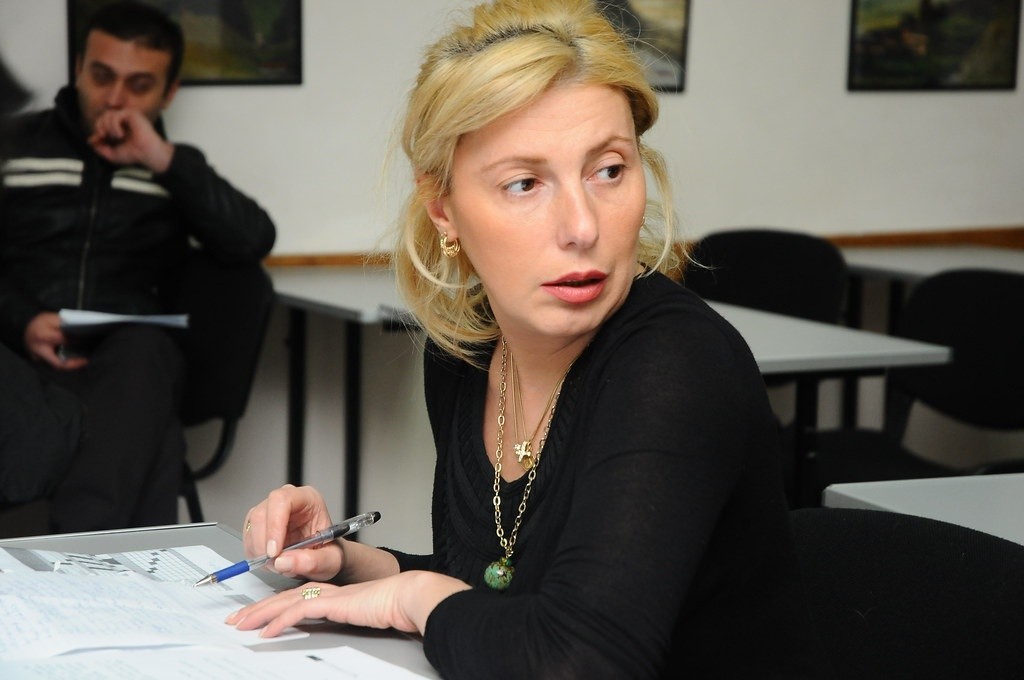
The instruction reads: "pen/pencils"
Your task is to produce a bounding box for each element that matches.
[56,346,71,367]
[193,509,382,592]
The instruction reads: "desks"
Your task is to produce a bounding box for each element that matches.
[0,513,446,679]
[823,473,1024,550]
[844,241,1024,336]
[376,283,954,512]
[265,268,428,543]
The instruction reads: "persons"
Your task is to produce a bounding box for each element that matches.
[0,2,276,534]
[224,0,814,680]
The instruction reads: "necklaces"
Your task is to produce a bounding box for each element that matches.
[484,335,595,590]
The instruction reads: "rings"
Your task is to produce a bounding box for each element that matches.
[302,587,321,600]
[246,520,251,531]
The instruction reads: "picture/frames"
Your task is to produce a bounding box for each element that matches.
[66,0,303,89]
[847,0,1022,92]
[631,0,690,93]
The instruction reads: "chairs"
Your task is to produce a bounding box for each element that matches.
[176,265,277,525]
[677,229,855,531]
[845,511,1024,680]
[867,265,1024,487]
